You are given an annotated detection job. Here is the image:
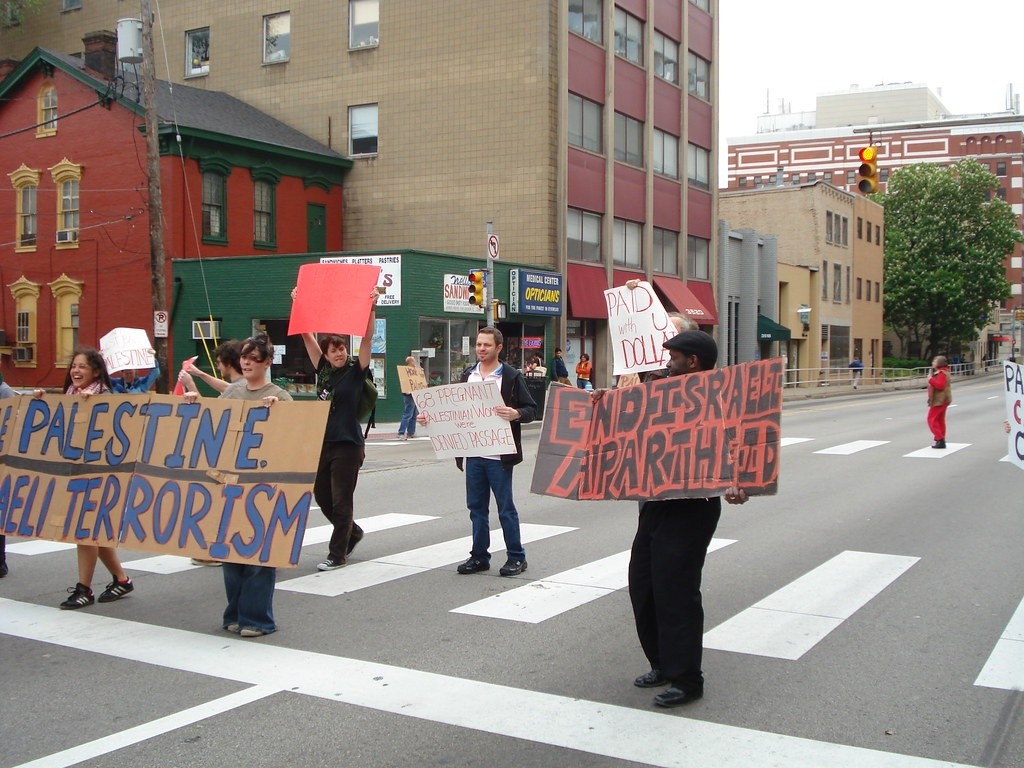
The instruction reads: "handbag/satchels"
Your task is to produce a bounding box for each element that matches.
[319,366,377,422]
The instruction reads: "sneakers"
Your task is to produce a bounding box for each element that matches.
[456,556,490,573]
[60,582,94,610]
[346,534,365,559]
[317,559,346,571]
[500,558,527,576]
[98,574,133,602]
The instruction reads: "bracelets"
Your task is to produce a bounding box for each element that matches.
[371,303,376,312]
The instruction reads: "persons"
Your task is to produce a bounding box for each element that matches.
[34,345,134,610]
[982,350,990,373]
[927,355,951,449]
[576,353,590,390]
[416,326,540,576]
[290,285,379,572]
[847,357,864,388]
[590,330,748,708]
[626,278,699,388]
[551,347,568,383]
[178,340,246,567]
[0,370,22,580]
[97,348,162,395]
[397,356,424,441]
[183,326,294,638]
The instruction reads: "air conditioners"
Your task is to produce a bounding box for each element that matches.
[56,231,75,243]
[191,320,221,341]
[17,348,33,362]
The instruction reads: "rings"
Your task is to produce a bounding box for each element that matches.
[730,497,735,500]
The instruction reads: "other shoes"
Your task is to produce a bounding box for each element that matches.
[240,628,263,636]
[932,439,947,449]
[191,558,222,567]
[0,564,8,577]
[227,623,239,631]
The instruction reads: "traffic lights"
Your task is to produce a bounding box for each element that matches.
[858,146,878,195]
[494,301,508,321]
[468,272,483,306]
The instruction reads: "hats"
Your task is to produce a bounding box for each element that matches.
[662,330,717,361]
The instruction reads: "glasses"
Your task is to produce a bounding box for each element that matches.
[214,360,223,366]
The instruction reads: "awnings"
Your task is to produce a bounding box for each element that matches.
[757,313,791,341]
[653,274,717,325]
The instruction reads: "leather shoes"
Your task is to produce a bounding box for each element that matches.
[654,671,703,707]
[634,668,671,688]
